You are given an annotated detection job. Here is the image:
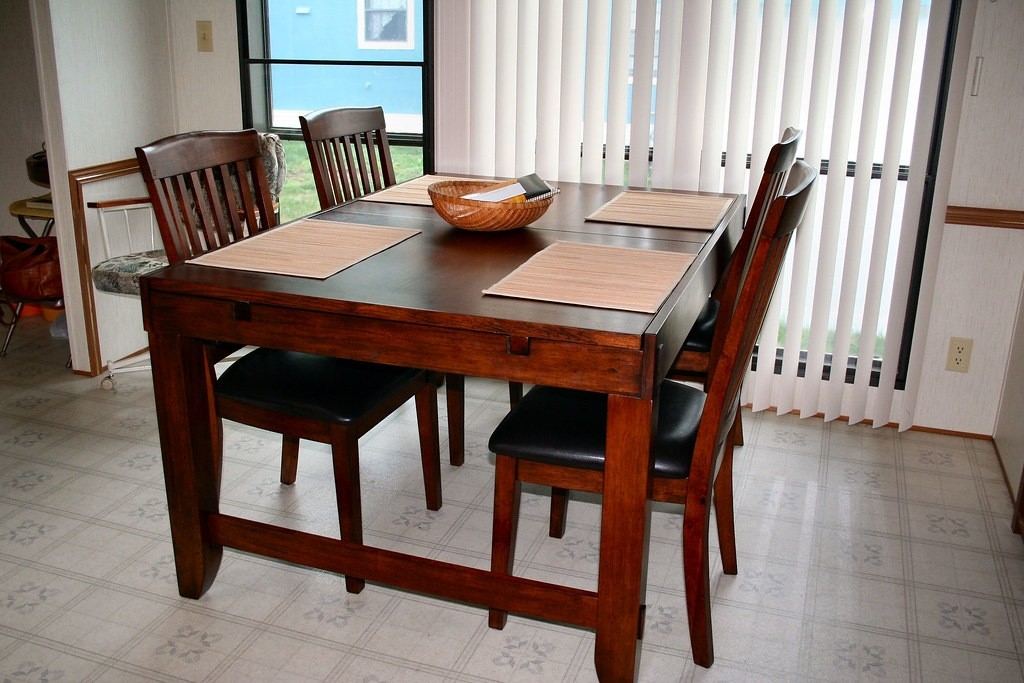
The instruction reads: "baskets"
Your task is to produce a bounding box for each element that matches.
[427,180,555,232]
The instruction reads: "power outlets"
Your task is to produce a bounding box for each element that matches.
[947,337,973,373]
[196,21,213,52]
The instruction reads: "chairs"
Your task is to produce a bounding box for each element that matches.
[299,106,522,465]
[68,131,287,389]
[670,126,800,447]
[488,162,817,668]
[135,131,442,594]
[0,151,76,368]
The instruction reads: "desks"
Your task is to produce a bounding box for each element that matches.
[139,173,747,683]
